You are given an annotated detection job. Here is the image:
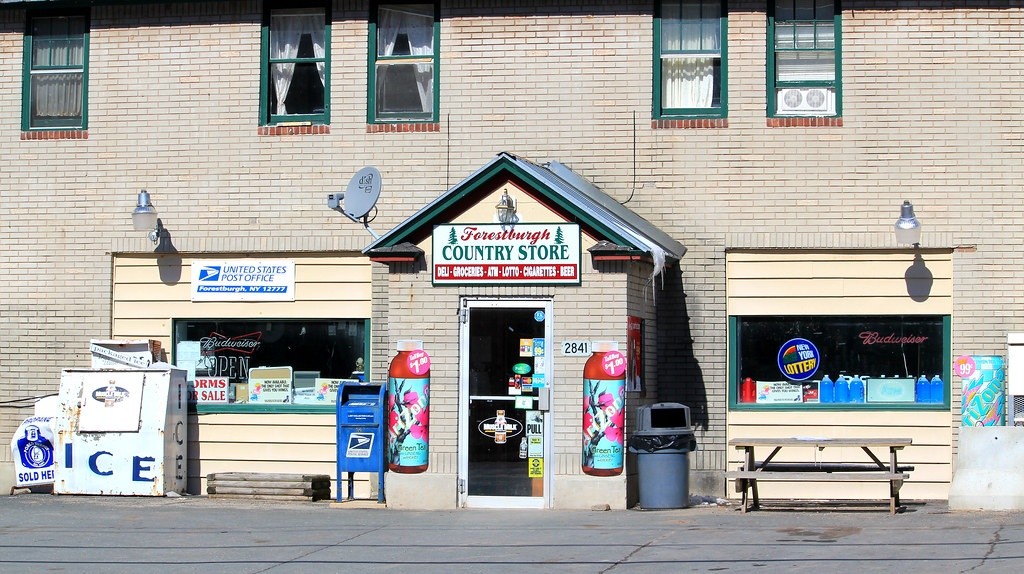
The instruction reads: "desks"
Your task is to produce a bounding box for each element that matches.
[727,437,913,516]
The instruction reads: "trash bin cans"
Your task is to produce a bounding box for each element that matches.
[628,403,693,511]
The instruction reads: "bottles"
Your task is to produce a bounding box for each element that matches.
[930,375,944,404]
[835,374,849,404]
[386,339,433,475]
[583,343,625,477]
[915,375,931,403]
[851,374,864,404]
[741,377,756,403]
[820,375,833,403]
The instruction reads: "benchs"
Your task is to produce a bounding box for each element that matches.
[724,470,910,495]
[740,461,915,474]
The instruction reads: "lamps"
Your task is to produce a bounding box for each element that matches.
[495,189,517,224]
[893,200,922,248]
[131,190,160,241]
